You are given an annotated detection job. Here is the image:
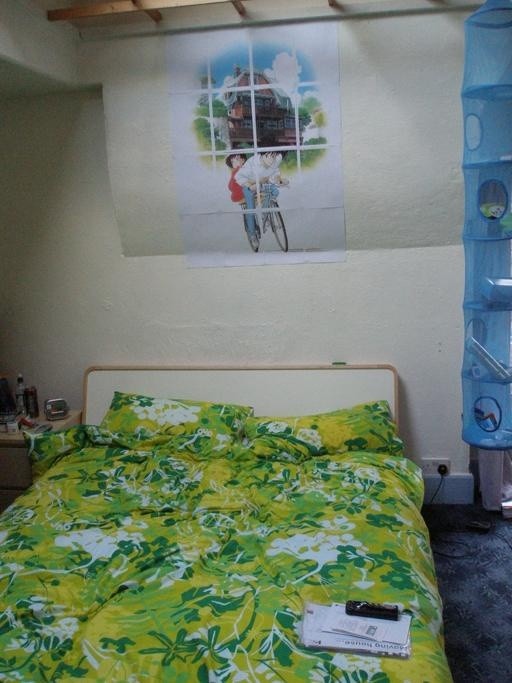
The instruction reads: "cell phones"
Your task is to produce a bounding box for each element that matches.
[347,600,399,621]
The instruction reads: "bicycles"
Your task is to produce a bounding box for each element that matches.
[239,180,289,252]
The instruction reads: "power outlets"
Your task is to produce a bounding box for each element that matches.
[419,456,451,476]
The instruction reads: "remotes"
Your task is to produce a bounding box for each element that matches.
[34,423,52,433]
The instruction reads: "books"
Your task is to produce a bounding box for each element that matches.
[301,600,412,660]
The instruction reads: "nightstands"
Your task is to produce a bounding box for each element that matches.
[0,411,83,511]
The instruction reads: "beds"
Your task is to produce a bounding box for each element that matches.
[0,362,451,683]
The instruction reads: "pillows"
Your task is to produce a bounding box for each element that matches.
[99,392,255,462]
[243,391,405,459]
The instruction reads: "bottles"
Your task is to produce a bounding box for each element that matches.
[14,373,27,416]
[24,384,39,419]
